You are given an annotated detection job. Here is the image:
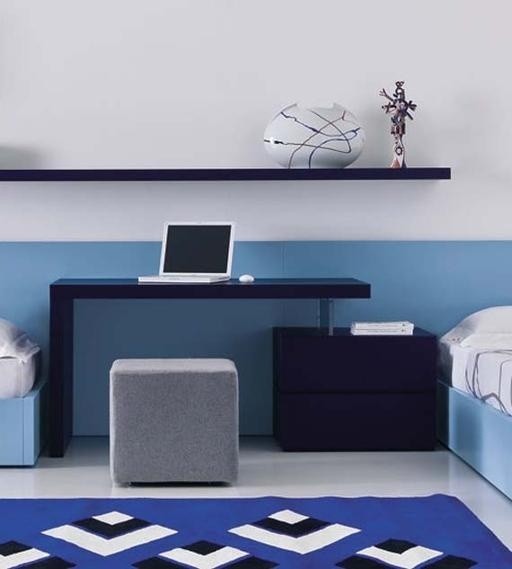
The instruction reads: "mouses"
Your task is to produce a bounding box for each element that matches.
[239,274,255,283]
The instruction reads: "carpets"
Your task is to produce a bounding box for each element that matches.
[0,493,512,568]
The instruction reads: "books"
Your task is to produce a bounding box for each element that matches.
[351,319,415,331]
[349,328,415,338]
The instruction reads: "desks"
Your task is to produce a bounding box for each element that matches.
[41,271,371,456]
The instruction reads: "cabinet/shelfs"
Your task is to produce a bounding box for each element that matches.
[270,324,438,452]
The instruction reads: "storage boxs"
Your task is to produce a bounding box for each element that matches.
[0,376,48,469]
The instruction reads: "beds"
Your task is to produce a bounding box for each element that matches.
[436,339,510,503]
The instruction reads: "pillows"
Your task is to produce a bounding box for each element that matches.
[440,303,512,350]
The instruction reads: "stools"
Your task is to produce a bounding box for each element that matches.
[108,354,241,488]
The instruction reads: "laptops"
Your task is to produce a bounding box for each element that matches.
[138,221,235,283]
